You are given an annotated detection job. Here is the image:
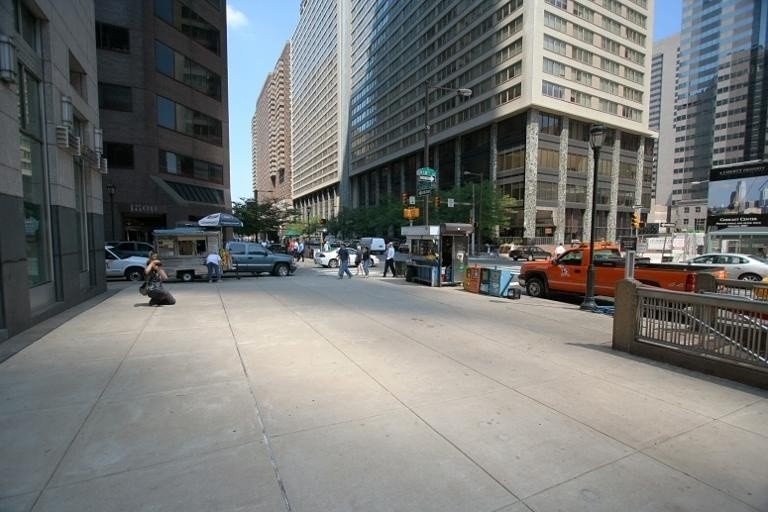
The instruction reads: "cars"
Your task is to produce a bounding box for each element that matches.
[508,246,551,261]
[753,277,768,299]
[105,246,152,282]
[498,244,516,257]
[678,253,768,288]
[104,242,154,257]
[249,234,410,256]
[315,247,380,268]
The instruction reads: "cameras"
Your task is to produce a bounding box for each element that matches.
[155,262,163,267]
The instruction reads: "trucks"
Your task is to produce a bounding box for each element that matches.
[152,228,297,281]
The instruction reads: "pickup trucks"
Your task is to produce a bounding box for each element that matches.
[517,244,727,309]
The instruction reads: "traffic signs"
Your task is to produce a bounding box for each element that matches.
[630,204,644,211]
[416,184,432,190]
[409,196,415,205]
[447,197,454,208]
[419,175,436,182]
[418,166,436,175]
[659,221,676,228]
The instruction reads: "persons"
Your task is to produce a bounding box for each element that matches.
[363,245,370,275]
[285,236,305,262]
[356,245,363,274]
[336,243,353,279]
[144,259,175,305]
[261,239,274,248]
[207,253,222,282]
[383,242,395,277]
[323,241,328,252]
[555,243,566,257]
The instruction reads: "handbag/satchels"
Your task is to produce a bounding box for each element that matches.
[140,283,148,295]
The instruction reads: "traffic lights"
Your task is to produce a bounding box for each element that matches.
[435,196,441,208]
[631,213,640,228]
[402,193,407,205]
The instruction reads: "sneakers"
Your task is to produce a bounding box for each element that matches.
[217,280,223,283]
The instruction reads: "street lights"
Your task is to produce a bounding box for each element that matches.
[254,189,275,242]
[305,206,311,234]
[463,171,483,257]
[105,182,116,242]
[423,79,471,226]
[578,123,607,313]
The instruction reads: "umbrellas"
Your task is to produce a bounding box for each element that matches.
[198,213,243,250]
